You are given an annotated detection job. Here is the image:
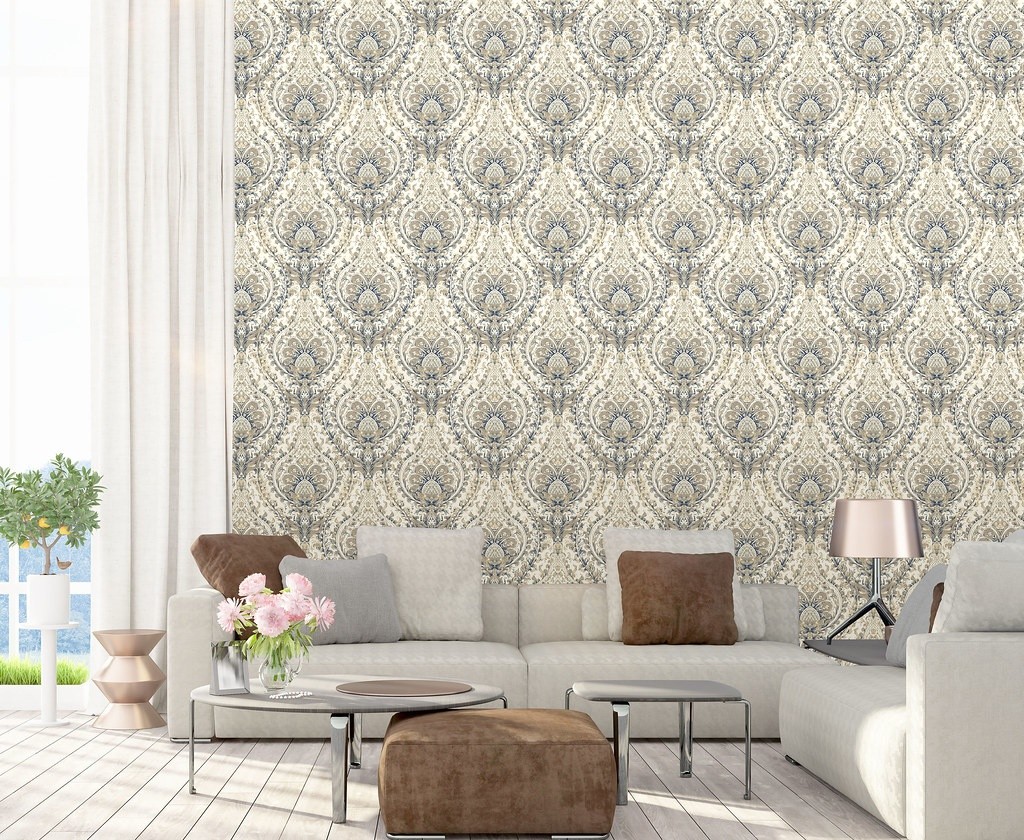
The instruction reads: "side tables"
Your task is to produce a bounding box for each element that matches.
[802,638,891,667]
[91,628,168,731]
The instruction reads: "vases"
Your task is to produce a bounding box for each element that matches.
[258,640,304,697]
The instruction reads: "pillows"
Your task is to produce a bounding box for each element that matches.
[616,550,741,647]
[277,553,404,646]
[356,524,484,642]
[602,527,749,643]
[884,564,949,671]
[929,539,1023,633]
[191,533,310,642]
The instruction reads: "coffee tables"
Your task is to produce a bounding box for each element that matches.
[187,673,510,824]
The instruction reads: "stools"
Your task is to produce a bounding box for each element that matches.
[563,679,752,806]
[376,706,618,839]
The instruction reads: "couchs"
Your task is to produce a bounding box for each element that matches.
[164,580,842,745]
[777,528,1023,840]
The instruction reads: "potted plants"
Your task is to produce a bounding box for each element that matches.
[0,454,108,627]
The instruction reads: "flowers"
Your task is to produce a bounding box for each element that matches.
[215,572,337,668]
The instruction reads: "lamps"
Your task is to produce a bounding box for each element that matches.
[826,499,925,646]
